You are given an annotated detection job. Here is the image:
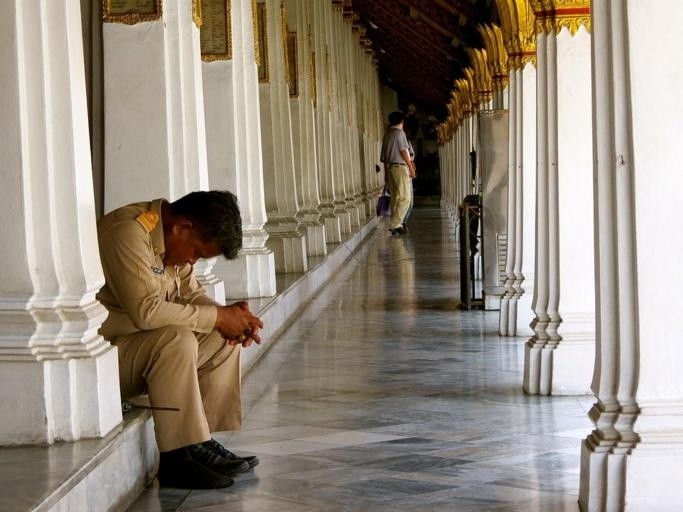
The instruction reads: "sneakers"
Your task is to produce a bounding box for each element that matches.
[390,223,410,235]
[189,439,259,475]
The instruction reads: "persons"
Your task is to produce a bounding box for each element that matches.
[380,126,415,233]
[96,191,263,488]
[384,112,417,236]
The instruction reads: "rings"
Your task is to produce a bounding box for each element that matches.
[245,329,251,336]
[239,336,246,342]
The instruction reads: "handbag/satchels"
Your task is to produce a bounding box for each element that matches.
[375,184,391,216]
